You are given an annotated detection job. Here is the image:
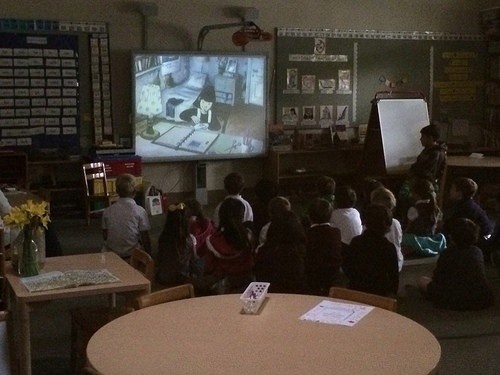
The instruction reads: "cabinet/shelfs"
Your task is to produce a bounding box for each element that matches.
[267,146,340,190]
[21,153,88,219]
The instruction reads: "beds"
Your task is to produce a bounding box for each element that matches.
[156,72,210,123]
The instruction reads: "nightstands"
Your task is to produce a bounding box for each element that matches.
[214,72,243,106]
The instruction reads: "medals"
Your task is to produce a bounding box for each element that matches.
[156,172,500,298]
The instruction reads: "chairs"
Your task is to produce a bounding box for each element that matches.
[82,162,120,226]
[136,283,194,308]
[329,287,398,312]
[70,248,153,373]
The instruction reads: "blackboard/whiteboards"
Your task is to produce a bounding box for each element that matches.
[376,98,431,176]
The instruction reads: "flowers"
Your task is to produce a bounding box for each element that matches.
[3,200,52,231]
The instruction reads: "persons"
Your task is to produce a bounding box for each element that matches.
[101,173,151,258]
[410,124,447,206]
[0,188,11,311]
[427,218,493,310]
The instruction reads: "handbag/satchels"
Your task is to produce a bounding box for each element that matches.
[134,186,163,209]
[144,188,163,216]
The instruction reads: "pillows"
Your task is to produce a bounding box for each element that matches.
[188,72,207,91]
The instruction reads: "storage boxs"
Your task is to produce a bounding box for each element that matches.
[92,155,141,210]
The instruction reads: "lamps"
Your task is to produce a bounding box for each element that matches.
[137,84,164,141]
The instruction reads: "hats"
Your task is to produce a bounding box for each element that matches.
[458,176,478,201]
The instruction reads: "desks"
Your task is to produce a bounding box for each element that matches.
[136,123,263,157]
[86,293,442,375]
[4,252,151,375]
[447,157,500,168]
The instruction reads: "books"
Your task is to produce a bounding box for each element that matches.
[20,268,122,293]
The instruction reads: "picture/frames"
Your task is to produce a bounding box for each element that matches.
[21,227,39,276]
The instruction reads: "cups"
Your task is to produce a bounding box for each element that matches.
[241,143,254,154]
